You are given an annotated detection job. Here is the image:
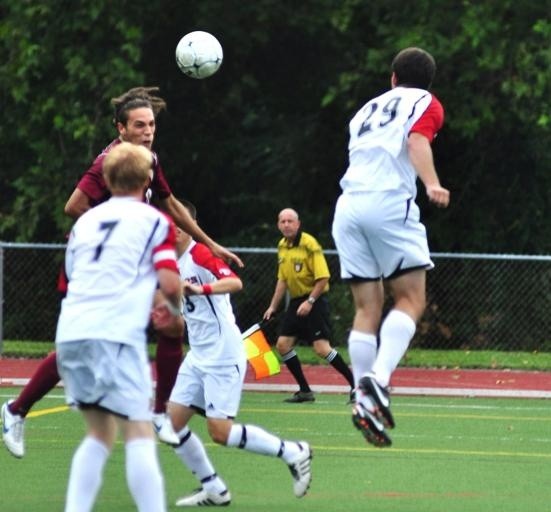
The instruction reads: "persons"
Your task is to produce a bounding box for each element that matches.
[330,46,453,448]
[1,86,245,460]
[154,198,313,507]
[55,140,183,512]
[263,206,355,403]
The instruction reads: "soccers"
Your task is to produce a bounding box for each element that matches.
[175,30,223,79]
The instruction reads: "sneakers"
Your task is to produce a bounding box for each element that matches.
[283,391,314,403]
[346,389,355,404]
[352,408,391,448]
[359,376,394,429]
[175,483,231,507]
[285,442,312,498]
[150,412,179,446]
[0,399,25,459]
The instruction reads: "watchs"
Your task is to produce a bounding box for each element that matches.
[308,298,316,304]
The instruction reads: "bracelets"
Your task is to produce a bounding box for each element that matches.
[202,285,212,295]
